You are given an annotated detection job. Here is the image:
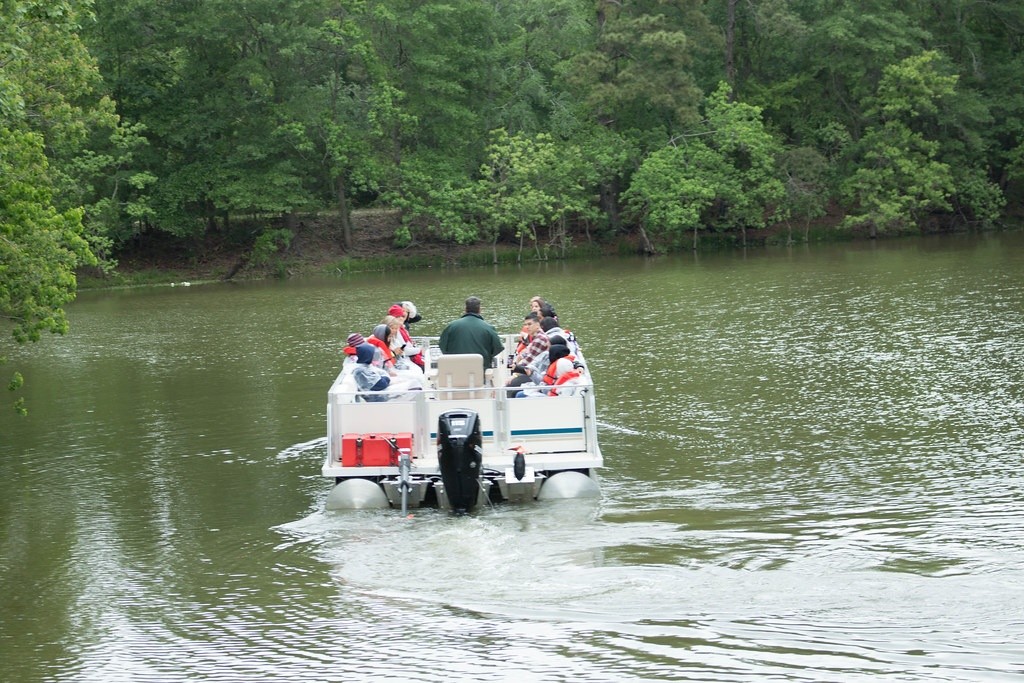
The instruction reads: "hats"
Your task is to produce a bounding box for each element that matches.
[533,300,544,308]
[388,304,405,318]
[556,357,573,375]
[347,333,364,347]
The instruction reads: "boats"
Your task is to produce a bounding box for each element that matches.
[320,332,609,513]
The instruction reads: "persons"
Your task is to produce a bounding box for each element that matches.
[439,296,505,385]
[506,296,575,398]
[346,300,425,402]
